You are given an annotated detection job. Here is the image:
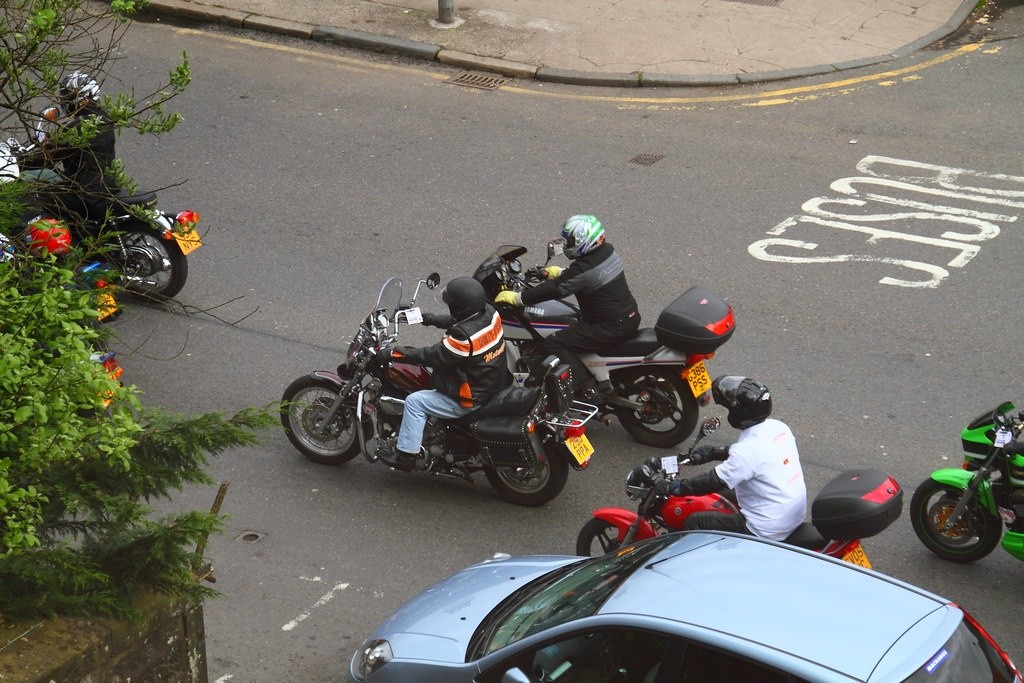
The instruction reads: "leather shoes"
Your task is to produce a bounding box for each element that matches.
[577,376,603,406]
[375,450,416,475]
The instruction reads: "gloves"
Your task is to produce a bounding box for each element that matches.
[669,478,682,495]
[495,291,524,308]
[371,348,390,371]
[691,444,714,464]
[422,312,433,325]
[541,265,566,279]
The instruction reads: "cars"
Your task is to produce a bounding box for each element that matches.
[349,529,1024,683]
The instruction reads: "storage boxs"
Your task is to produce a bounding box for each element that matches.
[654,287,736,355]
[812,469,904,542]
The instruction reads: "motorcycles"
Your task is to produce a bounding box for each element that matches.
[469,240,737,448]
[0,233,119,416]
[0,108,202,303]
[911,403,1024,561]
[281,273,599,506]
[576,416,902,576]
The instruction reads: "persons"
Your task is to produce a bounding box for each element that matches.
[12,219,95,358]
[654,375,806,541]
[495,214,641,405]
[17,71,114,215]
[373,277,514,474]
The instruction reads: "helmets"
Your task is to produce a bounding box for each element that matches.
[59,71,101,117]
[711,374,771,429]
[26,218,71,258]
[562,214,606,260]
[443,277,485,319]
